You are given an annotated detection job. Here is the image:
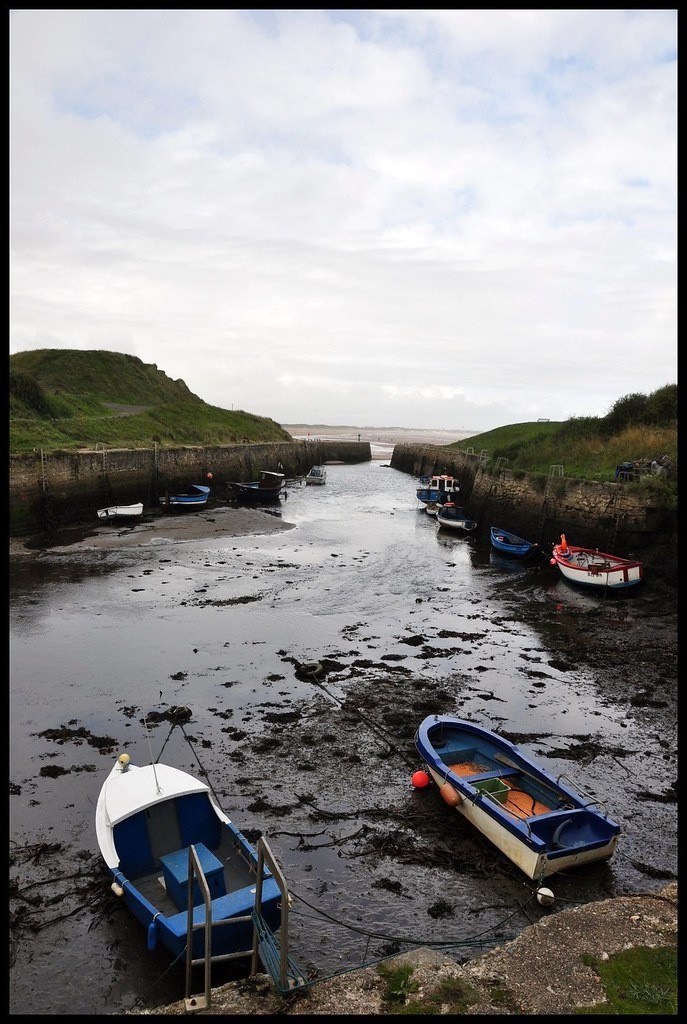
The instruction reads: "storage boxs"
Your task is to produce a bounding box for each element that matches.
[159,841,227,912]
[182,816,219,851]
[471,778,512,806]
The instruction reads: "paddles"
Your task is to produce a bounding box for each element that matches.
[494,752,567,800]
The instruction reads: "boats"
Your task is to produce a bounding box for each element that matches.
[158,484,210,510]
[417,472,479,532]
[489,526,540,557]
[415,715,620,883]
[550,533,643,591]
[97,502,143,523]
[226,471,286,500]
[96,751,315,997]
[306,463,327,484]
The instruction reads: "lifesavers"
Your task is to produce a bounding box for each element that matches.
[555,543,573,561]
[444,501,455,507]
[432,473,453,479]
